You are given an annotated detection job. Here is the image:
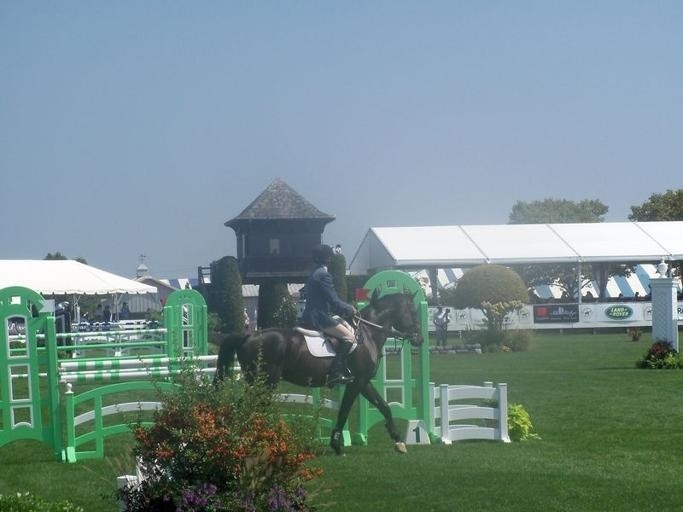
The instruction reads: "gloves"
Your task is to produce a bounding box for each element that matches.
[352,306,357,315]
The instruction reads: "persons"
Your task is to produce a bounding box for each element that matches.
[336,245,342,254]
[94,304,111,322]
[303,245,357,385]
[586,291,593,299]
[617,284,651,302]
[561,291,567,302]
[121,302,130,319]
[433,304,450,346]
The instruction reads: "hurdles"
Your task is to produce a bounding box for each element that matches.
[649,278,683,354]
[0,270,510,462]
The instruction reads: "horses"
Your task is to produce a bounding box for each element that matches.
[211,289,425,455]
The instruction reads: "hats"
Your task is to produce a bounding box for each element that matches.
[437,304,444,308]
[312,245,334,262]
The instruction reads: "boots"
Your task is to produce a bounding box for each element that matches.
[327,341,358,384]
